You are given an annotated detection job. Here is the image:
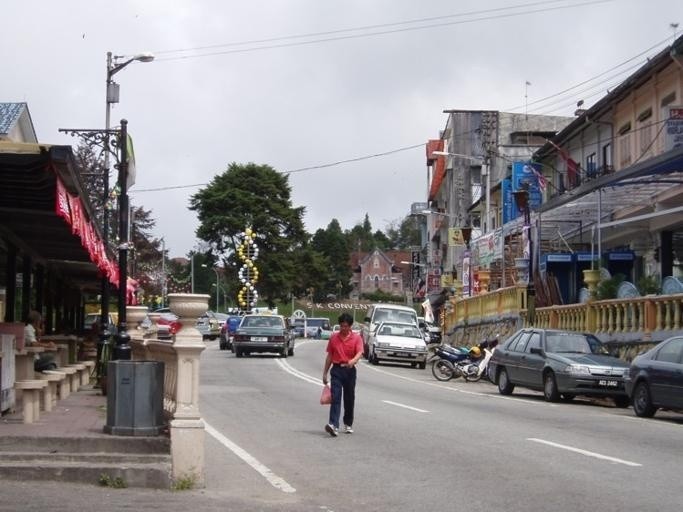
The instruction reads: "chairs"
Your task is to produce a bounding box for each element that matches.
[616,281,642,320]
[578,288,589,304]
[661,276,683,317]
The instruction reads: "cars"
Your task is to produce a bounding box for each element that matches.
[321,303,442,369]
[86,307,297,358]
[487,327,682,418]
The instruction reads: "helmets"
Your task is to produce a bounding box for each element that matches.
[469,347,481,357]
[231,309,238,315]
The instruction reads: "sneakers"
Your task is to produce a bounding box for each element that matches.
[343,424,354,433]
[325,423,339,436]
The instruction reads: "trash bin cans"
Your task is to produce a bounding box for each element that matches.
[103,360,165,435]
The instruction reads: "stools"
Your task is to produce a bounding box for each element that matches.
[13,360,95,424]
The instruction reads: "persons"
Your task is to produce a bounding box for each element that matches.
[24,311,58,372]
[323,314,364,436]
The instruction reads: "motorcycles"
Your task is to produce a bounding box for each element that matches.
[429,338,499,382]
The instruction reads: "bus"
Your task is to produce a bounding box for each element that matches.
[286,318,332,340]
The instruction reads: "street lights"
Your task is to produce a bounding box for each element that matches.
[422,151,491,271]
[155,237,233,312]
[92,51,154,389]
[400,260,428,294]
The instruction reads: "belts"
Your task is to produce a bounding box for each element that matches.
[334,363,347,367]
